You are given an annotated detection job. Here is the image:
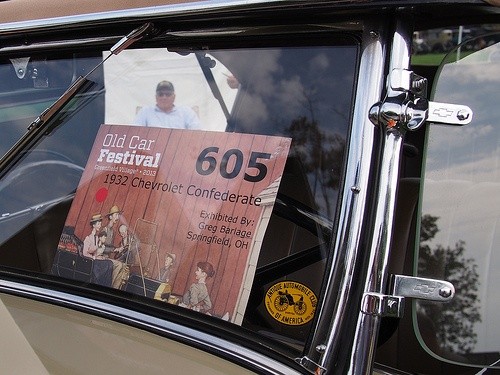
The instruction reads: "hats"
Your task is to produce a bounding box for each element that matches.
[157,81,174,92]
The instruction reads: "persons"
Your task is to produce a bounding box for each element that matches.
[137,79,200,130]
[226,74,240,89]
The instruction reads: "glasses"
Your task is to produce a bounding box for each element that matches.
[157,92,173,97]
